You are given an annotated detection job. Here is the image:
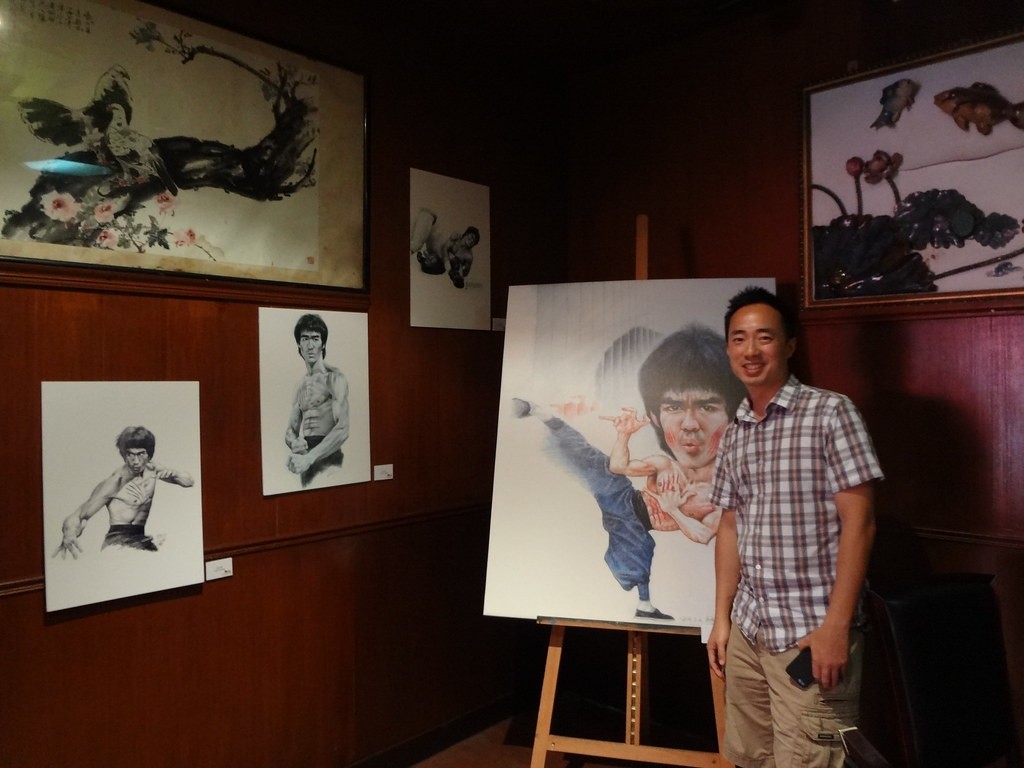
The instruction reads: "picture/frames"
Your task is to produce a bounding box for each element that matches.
[799,28,1024,325]
[0,0,372,312]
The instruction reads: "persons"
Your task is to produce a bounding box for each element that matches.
[703,286,884,767]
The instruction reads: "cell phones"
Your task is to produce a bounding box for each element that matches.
[785,646,813,688]
